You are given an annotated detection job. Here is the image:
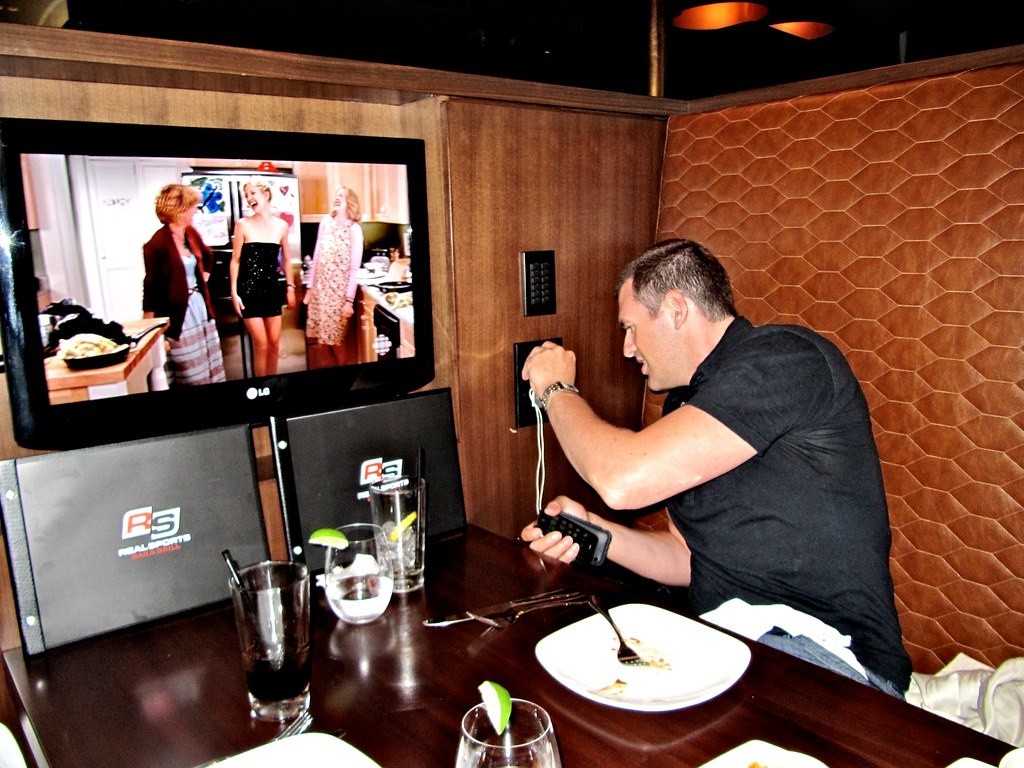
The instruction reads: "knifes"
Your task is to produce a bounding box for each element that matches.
[422,587,580,628]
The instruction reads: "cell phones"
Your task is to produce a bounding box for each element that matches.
[535,508,598,567]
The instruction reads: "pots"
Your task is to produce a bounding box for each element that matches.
[63,322,167,371]
[365,281,411,293]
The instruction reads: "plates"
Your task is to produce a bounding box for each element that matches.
[535,603,751,714]
[205,732,385,768]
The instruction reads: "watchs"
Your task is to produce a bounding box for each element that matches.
[540,381,582,412]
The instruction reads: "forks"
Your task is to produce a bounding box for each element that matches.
[192,710,313,768]
[586,594,650,667]
[466,599,588,628]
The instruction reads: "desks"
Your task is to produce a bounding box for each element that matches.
[41,316,171,405]
[1,521,1017,768]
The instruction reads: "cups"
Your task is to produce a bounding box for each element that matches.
[455,698,563,768]
[369,476,427,595]
[325,523,394,623]
[228,561,312,722]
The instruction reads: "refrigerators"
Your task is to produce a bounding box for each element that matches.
[180,170,307,381]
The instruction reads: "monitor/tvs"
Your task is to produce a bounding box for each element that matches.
[0,117,437,452]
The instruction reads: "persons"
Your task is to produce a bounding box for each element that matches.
[523,239,918,701]
[300,184,364,365]
[140,184,225,388]
[227,175,295,379]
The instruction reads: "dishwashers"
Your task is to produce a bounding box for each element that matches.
[373,304,400,361]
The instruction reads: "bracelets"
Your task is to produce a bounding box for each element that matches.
[344,299,355,305]
[286,283,297,289]
